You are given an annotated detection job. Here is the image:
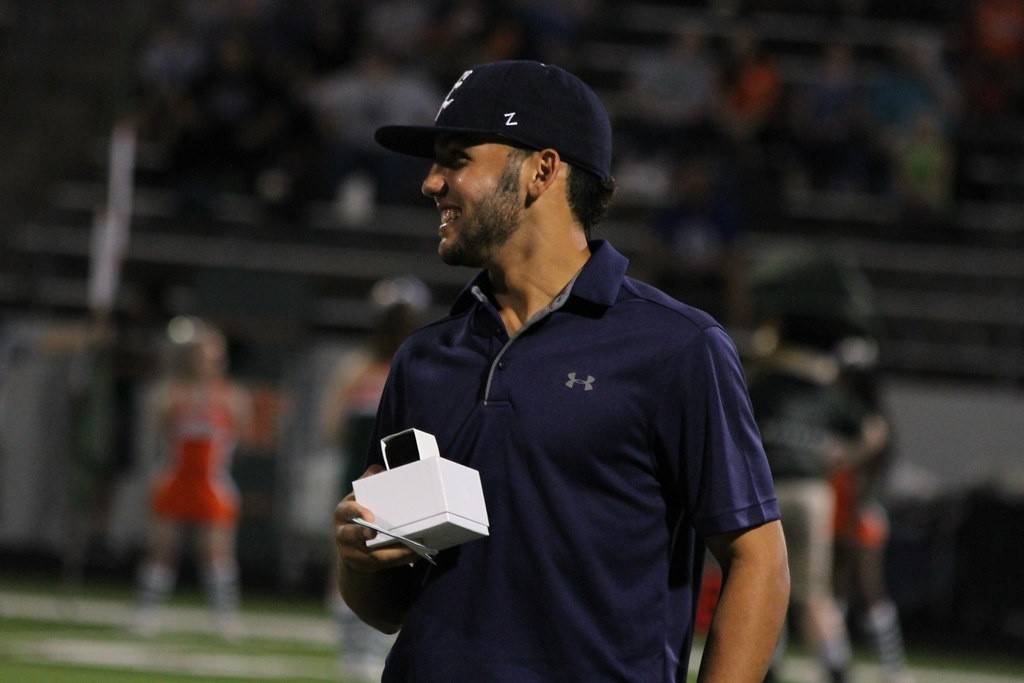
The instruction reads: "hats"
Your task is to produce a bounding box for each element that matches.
[373,61,612,181]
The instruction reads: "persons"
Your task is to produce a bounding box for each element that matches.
[332,60,791,683]
[161,0,1024,329]
[731,282,914,683]
[136,299,444,664]
